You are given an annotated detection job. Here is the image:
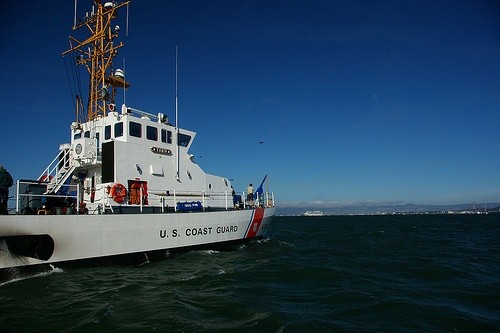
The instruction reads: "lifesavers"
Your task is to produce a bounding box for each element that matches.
[111,184,128,203]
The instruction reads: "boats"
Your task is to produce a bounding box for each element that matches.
[0,0,277,270]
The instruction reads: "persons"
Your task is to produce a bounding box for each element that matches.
[247,183,254,209]
[0,165,13,215]
